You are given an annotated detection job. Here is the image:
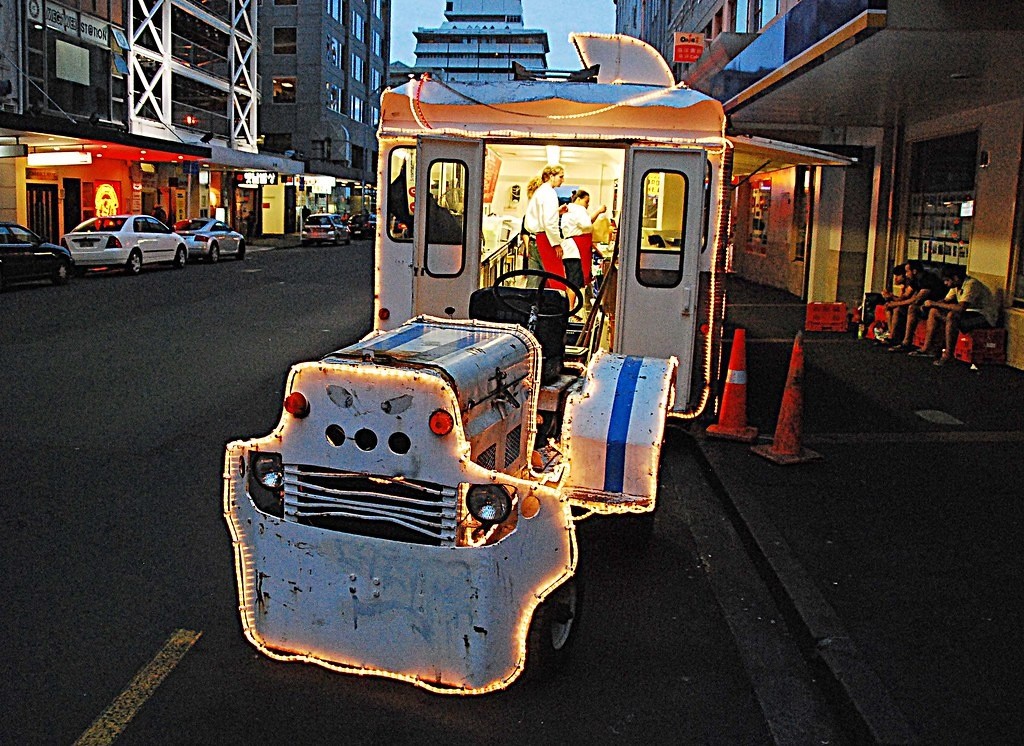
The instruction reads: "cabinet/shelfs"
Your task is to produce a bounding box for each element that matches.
[479,234,521,288]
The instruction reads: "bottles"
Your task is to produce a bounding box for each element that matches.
[608,220,616,243]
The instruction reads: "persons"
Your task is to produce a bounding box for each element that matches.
[317,207,326,214]
[302,205,312,224]
[334,207,341,215]
[561,189,608,322]
[521,166,569,291]
[152,203,167,225]
[877,260,949,354]
[244,211,256,246]
[908,263,1002,367]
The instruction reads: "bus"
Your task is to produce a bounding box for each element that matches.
[217,29,862,703]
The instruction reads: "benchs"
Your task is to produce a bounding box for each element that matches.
[875,304,1006,366]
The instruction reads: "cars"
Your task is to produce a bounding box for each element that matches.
[171,216,246,265]
[0,221,75,289]
[60,213,191,276]
[301,212,352,247]
[347,213,376,237]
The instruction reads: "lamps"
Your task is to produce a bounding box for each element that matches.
[200,131,213,144]
[980,151,988,169]
[88,111,100,125]
[32,99,44,117]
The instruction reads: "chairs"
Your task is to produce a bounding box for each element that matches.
[469,287,570,388]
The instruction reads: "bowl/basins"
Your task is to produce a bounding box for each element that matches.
[664,238,681,246]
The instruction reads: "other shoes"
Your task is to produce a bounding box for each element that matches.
[881,337,899,345]
[933,357,955,367]
[889,345,909,351]
[908,349,935,357]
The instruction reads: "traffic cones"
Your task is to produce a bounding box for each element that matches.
[750,330,824,466]
[704,327,760,443]
